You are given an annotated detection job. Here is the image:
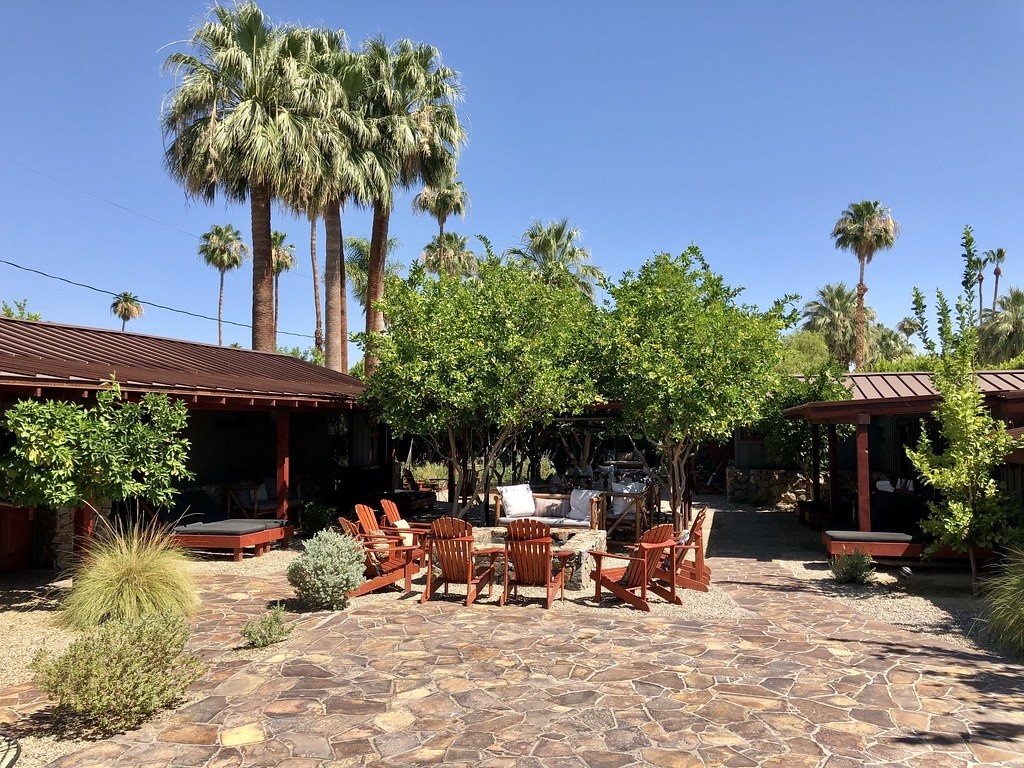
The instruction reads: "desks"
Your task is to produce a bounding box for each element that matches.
[429,478,447,492]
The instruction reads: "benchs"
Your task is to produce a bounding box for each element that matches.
[493,493,603,528]
[419,482,439,492]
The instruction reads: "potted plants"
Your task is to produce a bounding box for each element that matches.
[795,500,834,528]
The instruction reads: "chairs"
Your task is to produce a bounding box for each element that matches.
[355,503,426,569]
[381,498,436,566]
[585,476,711,612]
[418,516,500,605]
[456,469,478,506]
[498,519,575,612]
[820,511,999,573]
[403,469,436,492]
[337,516,421,598]
[141,483,295,561]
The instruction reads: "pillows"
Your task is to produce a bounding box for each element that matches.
[370,529,390,559]
[665,529,691,566]
[496,483,536,517]
[365,547,384,573]
[533,497,570,518]
[612,481,640,515]
[513,548,555,583]
[440,551,478,579]
[642,477,659,508]
[621,548,639,584]
[393,519,421,549]
[564,488,602,520]
